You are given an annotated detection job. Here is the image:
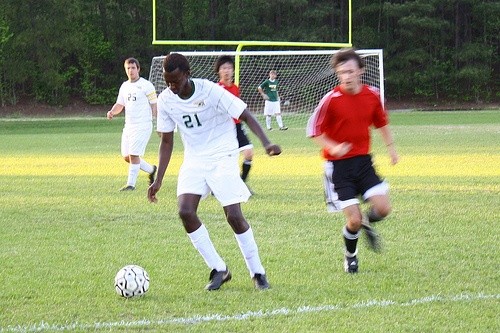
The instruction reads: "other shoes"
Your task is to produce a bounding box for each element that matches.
[281,126,289,130]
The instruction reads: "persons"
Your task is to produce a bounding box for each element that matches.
[306,49,398,274]
[148,53,282,292]
[214,56,254,181]
[107,57,158,192]
[258,70,288,131]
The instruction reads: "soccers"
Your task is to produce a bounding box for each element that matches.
[113,265,150,298]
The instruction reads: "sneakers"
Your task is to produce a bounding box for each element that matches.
[204,265,233,290]
[148,165,157,187]
[360,214,382,252]
[120,185,136,191]
[344,257,358,274]
[252,272,271,291]
